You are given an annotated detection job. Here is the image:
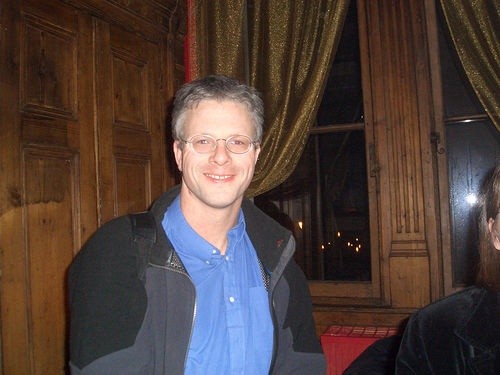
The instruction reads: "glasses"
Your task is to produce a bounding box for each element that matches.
[182,134,262,155]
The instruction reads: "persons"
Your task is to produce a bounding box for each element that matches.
[62,74,328,375]
[342,164,500,375]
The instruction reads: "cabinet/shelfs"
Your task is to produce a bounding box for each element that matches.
[0,0,190,374]
